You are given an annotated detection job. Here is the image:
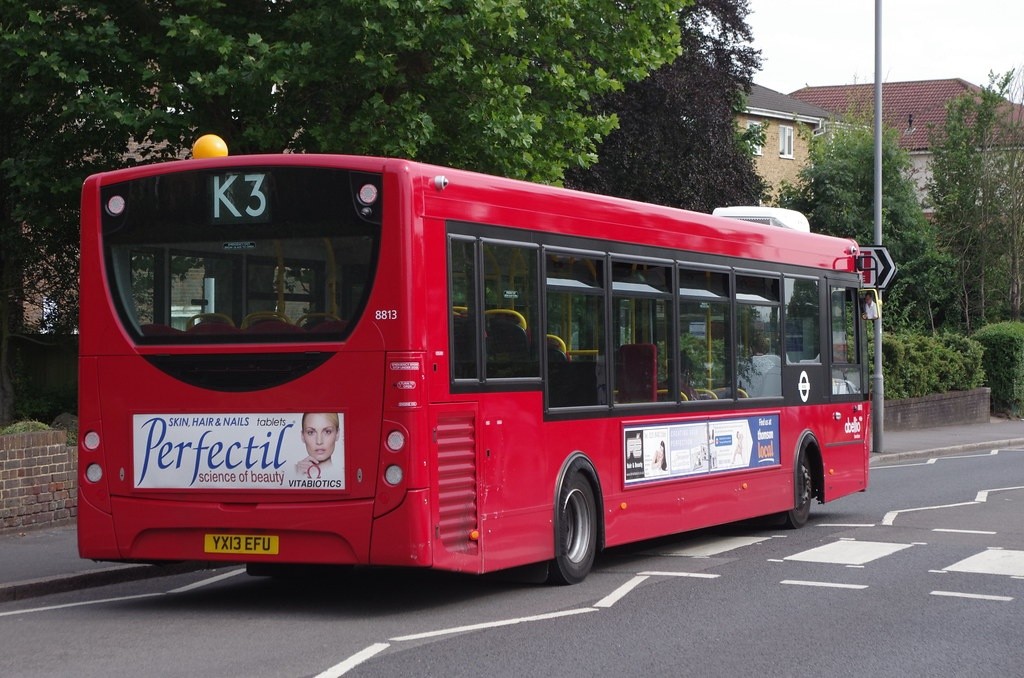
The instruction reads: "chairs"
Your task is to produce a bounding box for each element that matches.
[124,306,787,408]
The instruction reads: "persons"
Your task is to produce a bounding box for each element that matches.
[747,333,777,378]
[651,441,667,473]
[286,412,345,489]
[865,291,878,320]
[659,349,699,399]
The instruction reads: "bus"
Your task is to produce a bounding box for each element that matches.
[78,134,881,585]
